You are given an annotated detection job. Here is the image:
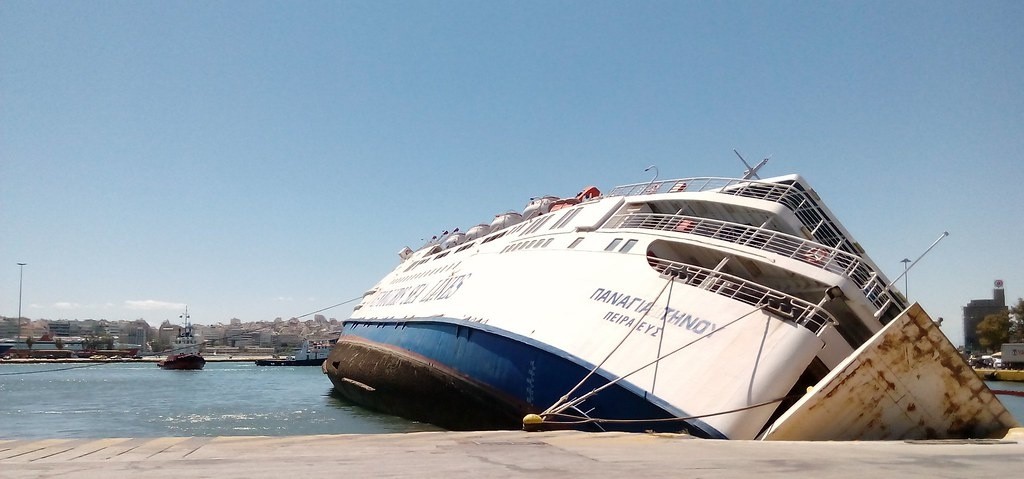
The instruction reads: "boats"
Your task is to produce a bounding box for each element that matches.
[255,338,338,366]
[322,149,950,440]
[157,352,207,370]
